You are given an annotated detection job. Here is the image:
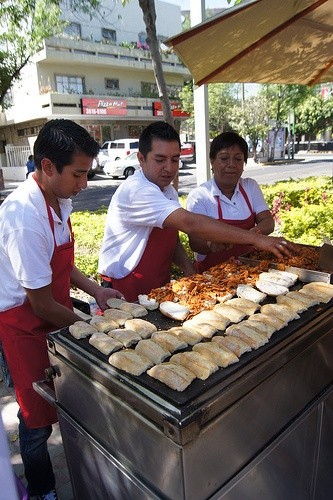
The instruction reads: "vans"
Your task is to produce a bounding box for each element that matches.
[96,139,140,167]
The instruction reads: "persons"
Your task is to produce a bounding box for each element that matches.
[26,155,36,172]
[98,120,300,303]
[185,132,275,275]
[0,119,126,500]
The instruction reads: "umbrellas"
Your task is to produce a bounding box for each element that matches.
[163,0,333,89]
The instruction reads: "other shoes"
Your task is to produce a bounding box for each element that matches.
[27,488,59,500]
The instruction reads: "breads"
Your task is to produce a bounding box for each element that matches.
[68,298,269,392]
[235,269,333,340]
[138,272,253,320]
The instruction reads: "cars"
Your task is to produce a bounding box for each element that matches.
[179,141,194,166]
[103,151,184,179]
